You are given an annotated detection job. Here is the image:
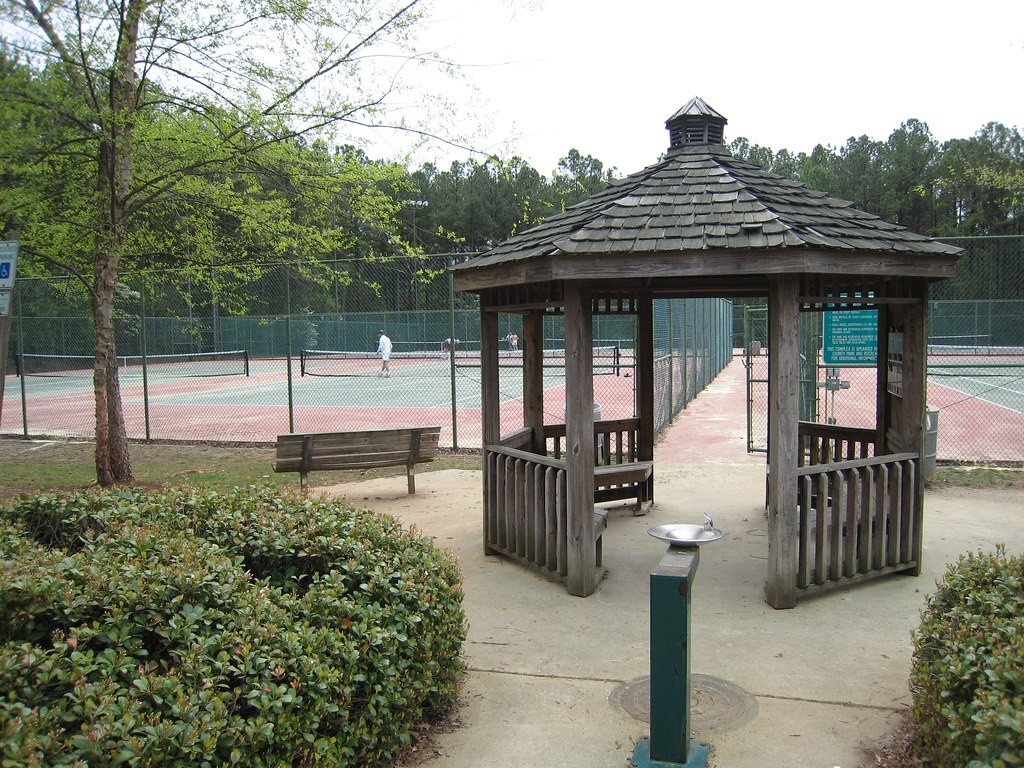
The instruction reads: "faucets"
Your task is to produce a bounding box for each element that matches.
[702,512,714,530]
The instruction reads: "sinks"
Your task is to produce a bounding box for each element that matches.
[647,523,725,547]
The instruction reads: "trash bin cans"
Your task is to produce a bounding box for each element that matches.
[925,405,940,483]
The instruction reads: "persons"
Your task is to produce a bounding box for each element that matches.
[374,328,393,378]
[505,332,519,352]
[442,338,460,352]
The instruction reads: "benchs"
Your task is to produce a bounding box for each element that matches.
[592,461,653,574]
[271,426,441,495]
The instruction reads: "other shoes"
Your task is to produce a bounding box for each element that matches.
[377,373,383,377]
[385,374,391,378]
